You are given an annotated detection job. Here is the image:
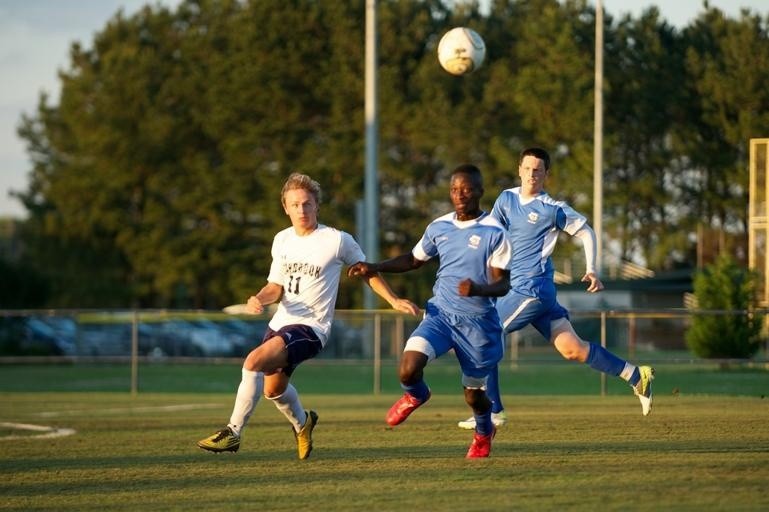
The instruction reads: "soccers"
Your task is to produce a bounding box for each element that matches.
[437,27,486,76]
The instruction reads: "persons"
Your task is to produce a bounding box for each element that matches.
[455,148,657,431]
[196,169,424,461]
[344,161,516,460]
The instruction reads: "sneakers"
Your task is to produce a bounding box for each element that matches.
[631,365,654,416]
[292,409,320,460]
[458,411,506,429]
[385,388,431,427]
[197,427,240,455]
[465,426,498,459]
